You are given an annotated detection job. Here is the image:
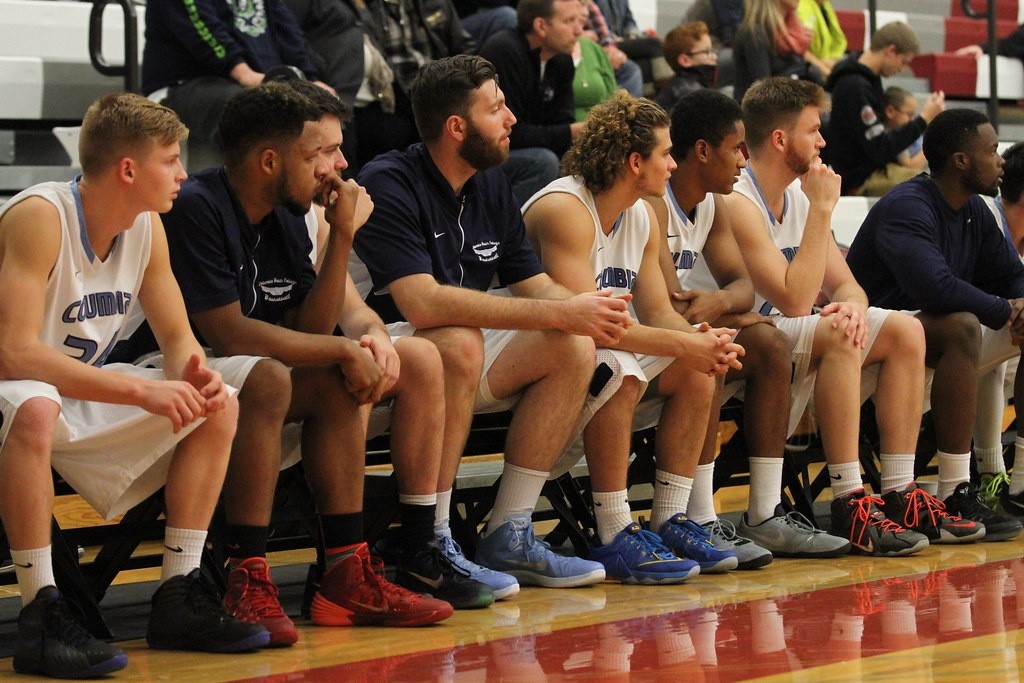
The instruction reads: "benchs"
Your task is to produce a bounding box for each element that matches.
[0,0,1024,652]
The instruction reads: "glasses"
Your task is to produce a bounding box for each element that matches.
[677,47,716,57]
[892,103,916,120]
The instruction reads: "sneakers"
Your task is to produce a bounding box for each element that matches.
[218,557,300,647]
[145,568,272,654]
[829,487,930,558]
[11,586,130,680]
[583,522,702,587]
[394,544,494,611]
[699,517,773,571]
[734,505,853,558]
[878,474,1024,545]
[637,512,738,574]
[302,554,456,628]
[431,537,521,600]
[304,561,451,618]
[472,512,607,588]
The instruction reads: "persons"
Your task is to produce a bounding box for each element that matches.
[0,93,270,675]
[142,1,947,202]
[523,91,745,584]
[643,91,851,568]
[847,111,1024,544]
[725,79,986,556]
[346,53,633,598]
[294,78,493,609]
[111,81,449,641]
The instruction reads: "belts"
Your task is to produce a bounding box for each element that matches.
[352,101,378,115]
